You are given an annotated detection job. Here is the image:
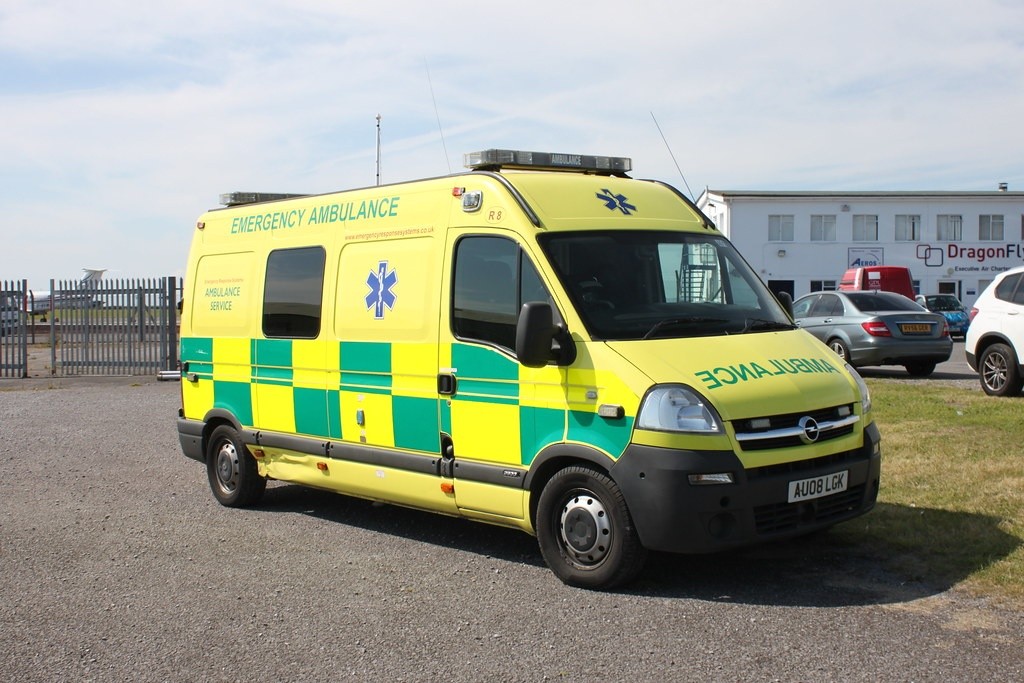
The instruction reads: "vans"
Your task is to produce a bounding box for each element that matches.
[835,264,917,302]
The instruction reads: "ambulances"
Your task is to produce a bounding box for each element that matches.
[174,146,883,592]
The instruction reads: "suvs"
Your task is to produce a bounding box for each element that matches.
[963,265,1024,398]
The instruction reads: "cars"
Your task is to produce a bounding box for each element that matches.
[915,293,969,338]
[790,289,954,377]
